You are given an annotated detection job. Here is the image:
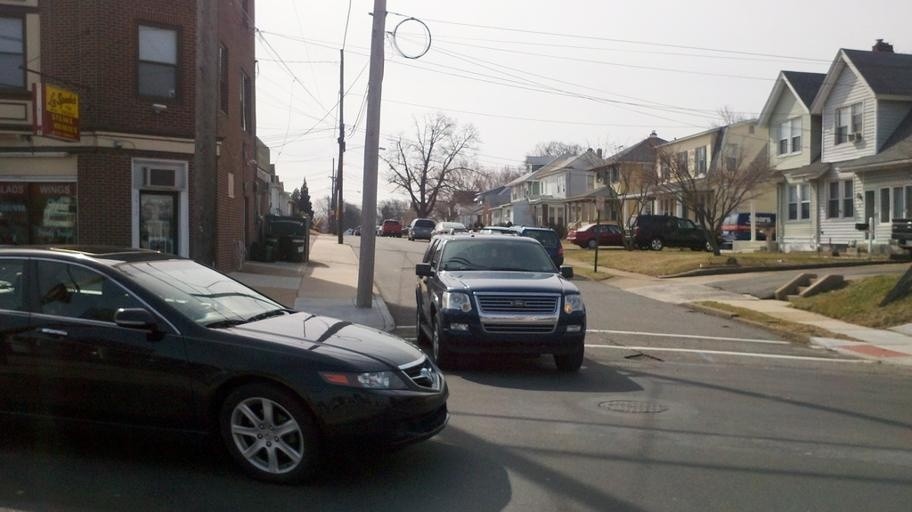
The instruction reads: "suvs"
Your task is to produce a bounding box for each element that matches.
[407,231,587,383]
[624,213,720,251]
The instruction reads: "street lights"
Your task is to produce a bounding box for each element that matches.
[337,144,386,244]
[342,188,361,195]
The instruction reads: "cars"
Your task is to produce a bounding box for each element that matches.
[571,224,631,252]
[0,240,448,487]
[345,218,565,266]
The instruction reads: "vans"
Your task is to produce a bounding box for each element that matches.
[722,211,776,248]
